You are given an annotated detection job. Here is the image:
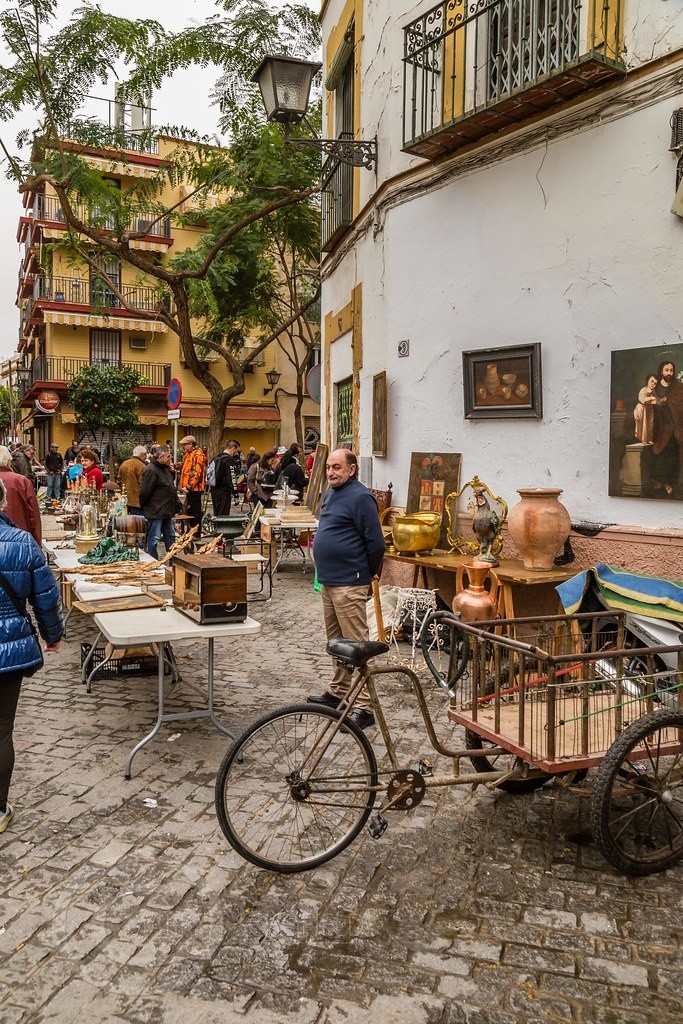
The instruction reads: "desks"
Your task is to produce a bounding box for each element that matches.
[41,539,77,566]
[384,549,582,702]
[259,508,319,578]
[54,550,262,781]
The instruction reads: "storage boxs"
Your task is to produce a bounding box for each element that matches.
[65,580,82,611]
[81,642,171,680]
[241,541,277,574]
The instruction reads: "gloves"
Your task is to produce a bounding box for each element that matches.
[234,497,240,506]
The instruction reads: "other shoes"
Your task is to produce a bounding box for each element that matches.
[214,543,225,552]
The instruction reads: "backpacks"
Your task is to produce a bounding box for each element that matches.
[205,453,230,486]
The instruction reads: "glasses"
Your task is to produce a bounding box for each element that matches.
[28,450,34,454]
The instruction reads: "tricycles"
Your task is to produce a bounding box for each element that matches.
[215,610,682,878]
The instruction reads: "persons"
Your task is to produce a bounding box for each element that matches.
[205,440,241,545]
[139,446,182,566]
[0,445,43,547]
[78,449,103,493]
[118,446,148,549]
[307,448,386,732]
[0,479,66,834]
[170,435,206,542]
[8,435,316,539]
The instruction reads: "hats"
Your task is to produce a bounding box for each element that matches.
[276,446,288,454]
[51,442,59,447]
[179,435,196,443]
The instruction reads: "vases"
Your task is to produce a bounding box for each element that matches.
[213,515,249,554]
[508,488,570,571]
[452,561,498,650]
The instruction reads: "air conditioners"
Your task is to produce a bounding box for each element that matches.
[134,217,159,235]
[52,208,77,223]
[130,337,147,348]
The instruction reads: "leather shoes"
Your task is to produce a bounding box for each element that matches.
[306,691,347,710]
[339,708,376,734]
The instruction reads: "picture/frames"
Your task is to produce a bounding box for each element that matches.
[372,370,387,458]
[461,342,542,418]
[72,592,169,614]
[304,443,328,515]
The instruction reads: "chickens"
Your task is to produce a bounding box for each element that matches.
[468,486,505,562]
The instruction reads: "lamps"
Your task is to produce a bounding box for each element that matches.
[264,367,282,396]
[250,44,379,172]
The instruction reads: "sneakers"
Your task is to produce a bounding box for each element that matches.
[0,803,15,833]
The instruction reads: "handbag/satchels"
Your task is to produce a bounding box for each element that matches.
[0,572,44,679]
[273,465,299,490]
[246,464,260,499]
[172,495,183,514]
